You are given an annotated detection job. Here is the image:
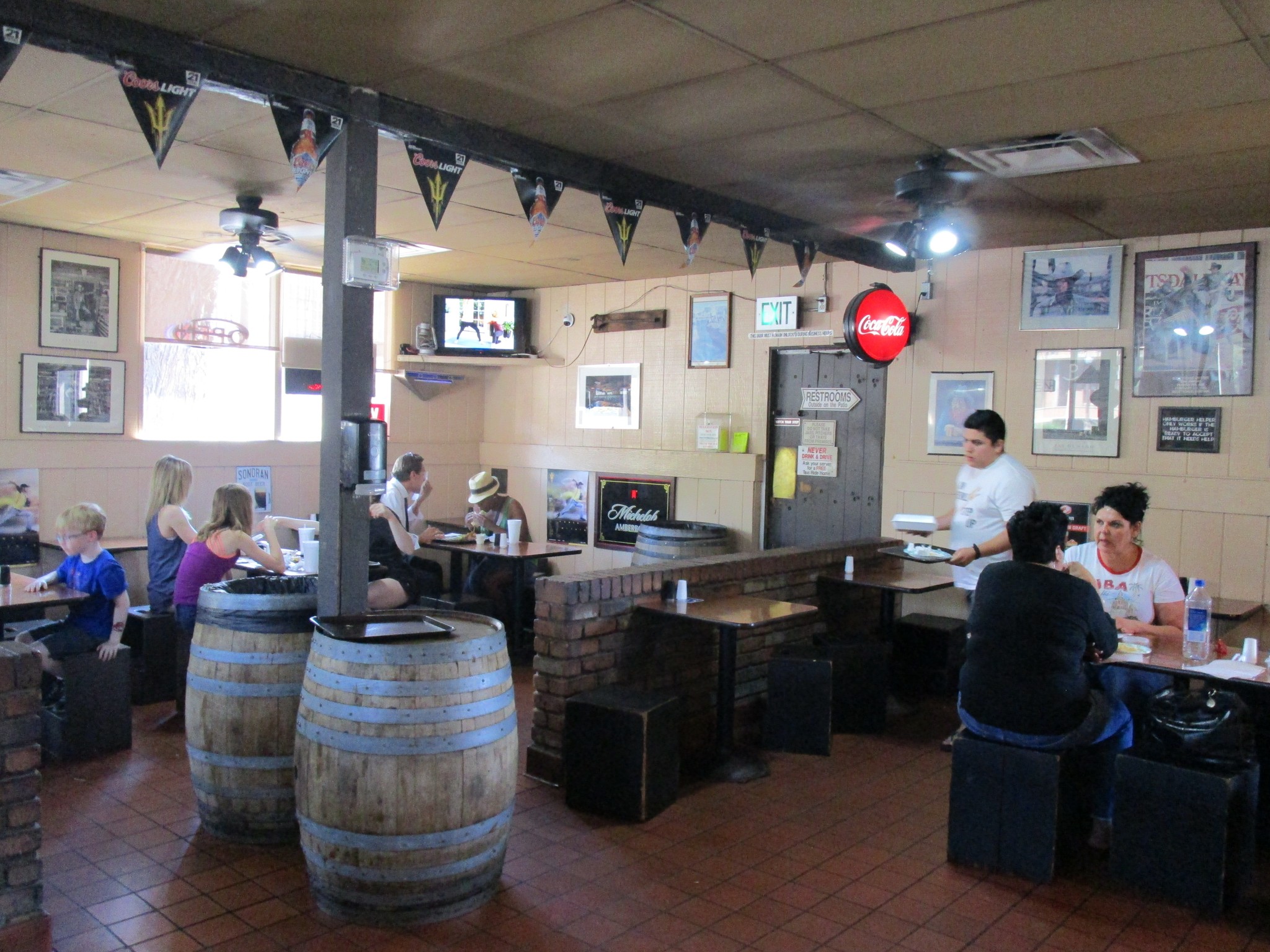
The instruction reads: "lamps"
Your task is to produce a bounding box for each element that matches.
[885,156,972,261]
[219,197,291,278]
[415,322,438,356]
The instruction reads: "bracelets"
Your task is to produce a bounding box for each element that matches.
[973,543,981,560]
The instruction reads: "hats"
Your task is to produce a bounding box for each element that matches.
[468,472,499,504]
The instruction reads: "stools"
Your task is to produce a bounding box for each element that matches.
[4,604,195,765]
[893,612,966,700]
[566,685,682,822]
[761,630,889,754]
[945,739,1264,916]
[421,588,535,651]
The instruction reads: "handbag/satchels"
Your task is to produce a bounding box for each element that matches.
[1146,687,1257,773]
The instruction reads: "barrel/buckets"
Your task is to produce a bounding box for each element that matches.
[631,520,732,567]
[294,607,519,927]
[184,576,318,844]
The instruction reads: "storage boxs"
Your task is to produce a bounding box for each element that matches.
[342,235,402,292]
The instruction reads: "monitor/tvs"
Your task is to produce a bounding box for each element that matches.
[434,294,527,357]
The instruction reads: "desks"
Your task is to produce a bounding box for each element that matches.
[640,590,819,787]
[425,517,480,574]
[1109,633,1270,690]
[234,555,389,579]
[1209,596,1262,622]
[420,540,583,667]
[820,567,955,718]
[40,537,149,557]
[0,571,91,642]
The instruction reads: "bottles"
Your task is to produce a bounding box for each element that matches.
[1242,637,1257,663]
[676,580,687,599]
[845,556,854,573]
[494,533,507,547]
[1182,580,1212,659]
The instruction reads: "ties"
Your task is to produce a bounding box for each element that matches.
[405,497,409,532]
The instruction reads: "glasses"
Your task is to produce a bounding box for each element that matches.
[57,530,91,543]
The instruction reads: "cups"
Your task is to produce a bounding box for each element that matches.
[0,562,11,587]
[507,519,522,544]
[476,534,485,544]
[302,541,319,573]
[255,486,266,508]
[299,528,315,553]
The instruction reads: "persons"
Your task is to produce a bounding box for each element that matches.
[256,493,423,611]
[465,472,533,631]
[898,409,1038,751]
[454,301,482,344]
[73,284,85,327]
[1032,262,1095,317]
[380,452,445,602]
[173,483,286,628]
[487,314,503,346]
[14,503,131,708]
[957,505,1132,854]
[144,456,200,614]
[0,480,37,533]
[1063,482,1187,698]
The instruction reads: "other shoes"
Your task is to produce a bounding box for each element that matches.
[940,725,967,750]
[40,673,64,707]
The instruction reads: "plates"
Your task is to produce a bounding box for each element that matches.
[904,548,952,560]
[1115,643,1151,654]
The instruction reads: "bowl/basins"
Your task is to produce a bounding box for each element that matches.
[890,514,939,531]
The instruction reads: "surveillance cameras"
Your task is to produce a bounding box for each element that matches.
[563,313,574,327]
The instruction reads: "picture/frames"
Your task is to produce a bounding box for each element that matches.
[927,370,995,455]
[1133,241,1258,399]
[1019,245,1126,331]
[19,245,128,435]
[574,291,731,429]
[1031,348,1124,459]
